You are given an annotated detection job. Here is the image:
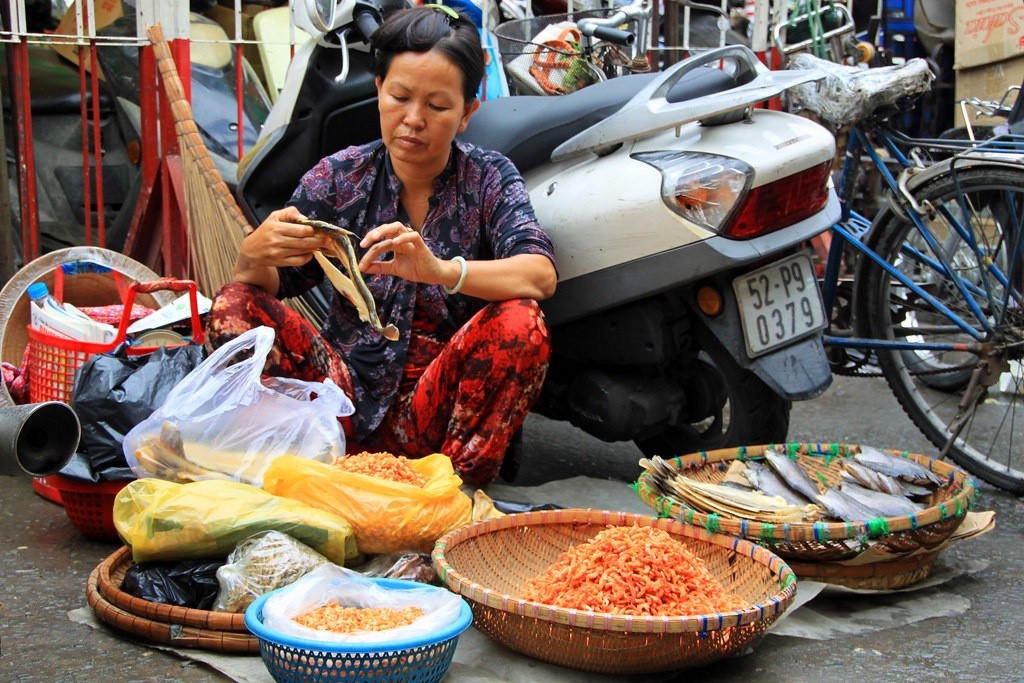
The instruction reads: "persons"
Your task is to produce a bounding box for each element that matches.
[205,7,559,497]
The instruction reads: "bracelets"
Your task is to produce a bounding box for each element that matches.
[443,255,467,294]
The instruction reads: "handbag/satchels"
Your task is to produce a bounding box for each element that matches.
[121,325,355,490]
[505,21,622,96]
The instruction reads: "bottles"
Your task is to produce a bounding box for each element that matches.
[27,282,62,310]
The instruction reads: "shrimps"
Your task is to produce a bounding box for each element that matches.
[291,450,749,634]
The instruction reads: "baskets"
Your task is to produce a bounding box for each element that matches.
[244,576,473,683]
[788,548,951,593]
[86,528,436,657]
[430,508,798,675]
[97,543,245,634]
[634,440,974,567]
[42,473,132,537]
[489,8,634,97]
[28,262,206,508]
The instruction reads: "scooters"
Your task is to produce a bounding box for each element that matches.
[1,0,1023,497]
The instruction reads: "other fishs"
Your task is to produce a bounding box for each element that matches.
[283,219,401,343]
[635,442,948,523]
[133,419,271,489]
